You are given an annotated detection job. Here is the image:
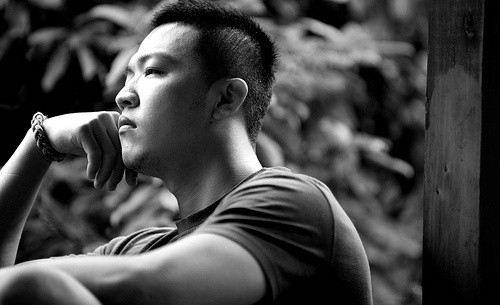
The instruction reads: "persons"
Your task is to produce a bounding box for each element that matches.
[0,1,374,304]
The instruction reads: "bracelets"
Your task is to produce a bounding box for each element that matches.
[31,111,66,163]
